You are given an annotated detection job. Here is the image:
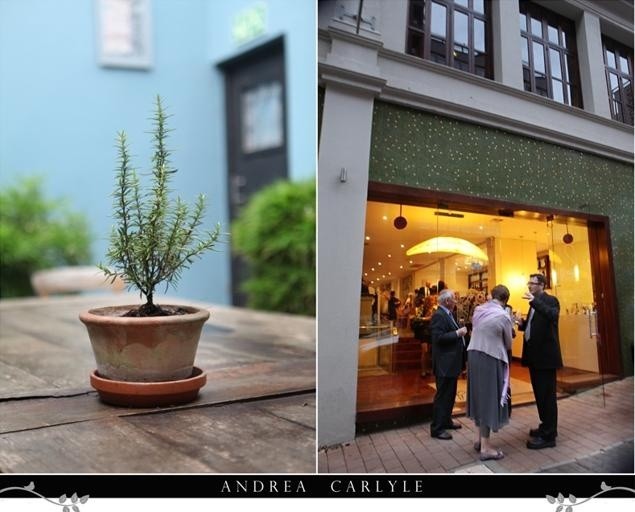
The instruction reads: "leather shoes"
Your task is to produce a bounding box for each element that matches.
[437,423,461,439]
[527,428,557,449]
[474,442,504,461]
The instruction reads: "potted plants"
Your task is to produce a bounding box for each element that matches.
[78,94,230,409]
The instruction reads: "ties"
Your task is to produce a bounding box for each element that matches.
[524,308,535,342]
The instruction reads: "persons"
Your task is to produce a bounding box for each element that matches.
[467,285,514,459]
[404,280,451,379]
[388,289,401,331]
[428,290,470,439]
[514,273,563,449]
